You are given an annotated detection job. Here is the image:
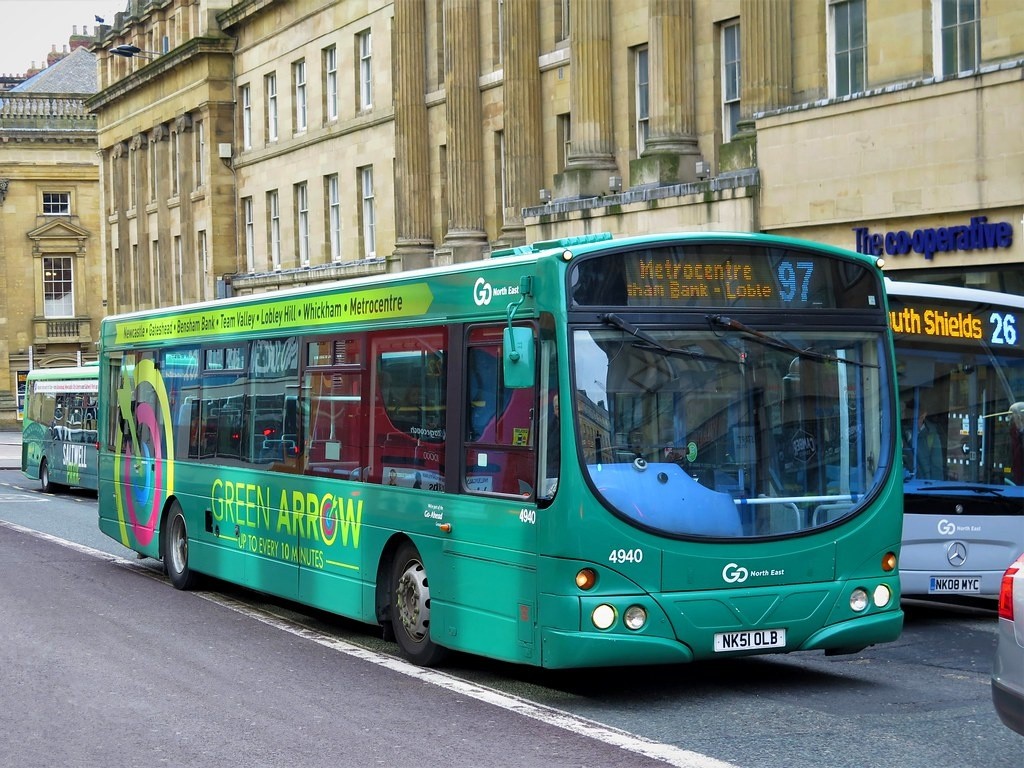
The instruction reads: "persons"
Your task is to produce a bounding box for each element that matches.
[901,397,944,481]
[547,394,558,476]
[398,383,429,426]
[1006,401,1024,485]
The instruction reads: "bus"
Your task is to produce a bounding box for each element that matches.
[870,278,1024,604]
[20,360,98,493]
[99,231,905,673]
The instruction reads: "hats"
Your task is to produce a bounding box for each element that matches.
[905,399,927,418]
